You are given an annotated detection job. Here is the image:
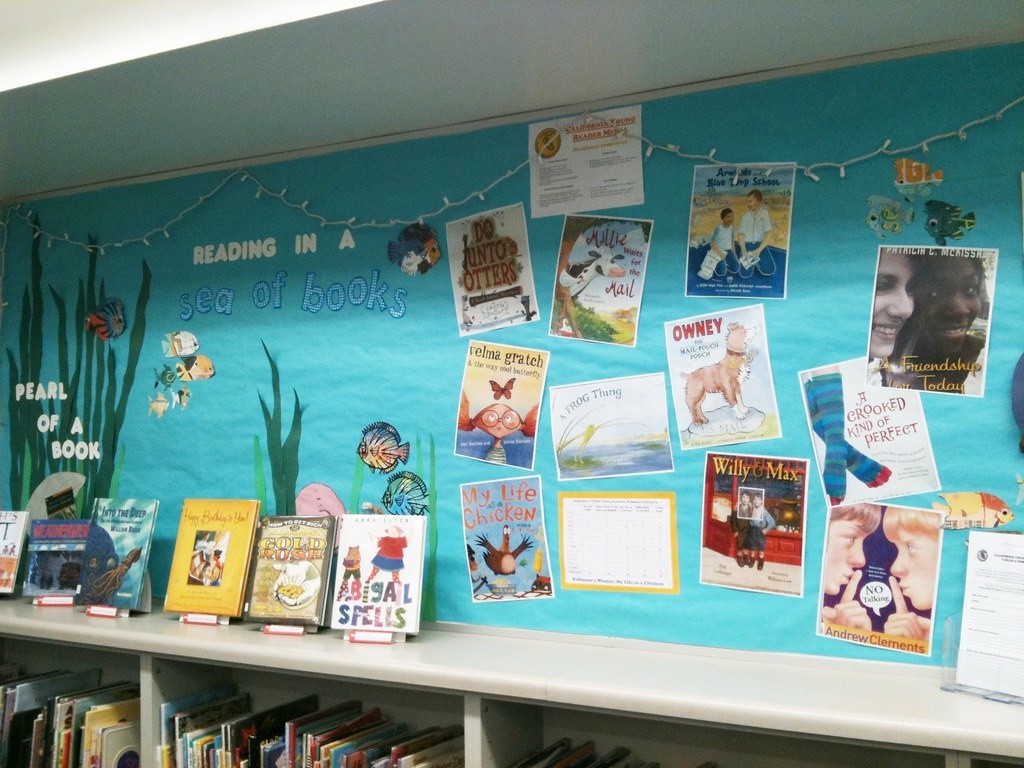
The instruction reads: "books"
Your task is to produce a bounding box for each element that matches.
[157,683,463,768]
[163,497,261,617]
[0,510,29,596]
[331,513,427,635]
[243,515,339,627]
[0,657,141,768]
[21,519,90,598]
[74,498,160,611]
[507,738,719,768]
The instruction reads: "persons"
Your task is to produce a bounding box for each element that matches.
[730,491,776,570]
[865,247,992,396]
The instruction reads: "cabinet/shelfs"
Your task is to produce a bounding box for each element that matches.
[1,581,1024,768]
